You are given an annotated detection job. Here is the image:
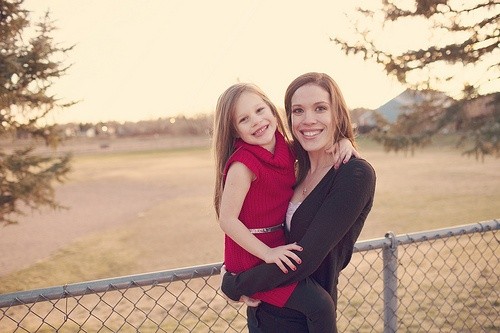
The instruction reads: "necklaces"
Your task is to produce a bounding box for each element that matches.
[302,161,332,195]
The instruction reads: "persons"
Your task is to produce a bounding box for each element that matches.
[218,72,376,333]
[212,82,361,333]
